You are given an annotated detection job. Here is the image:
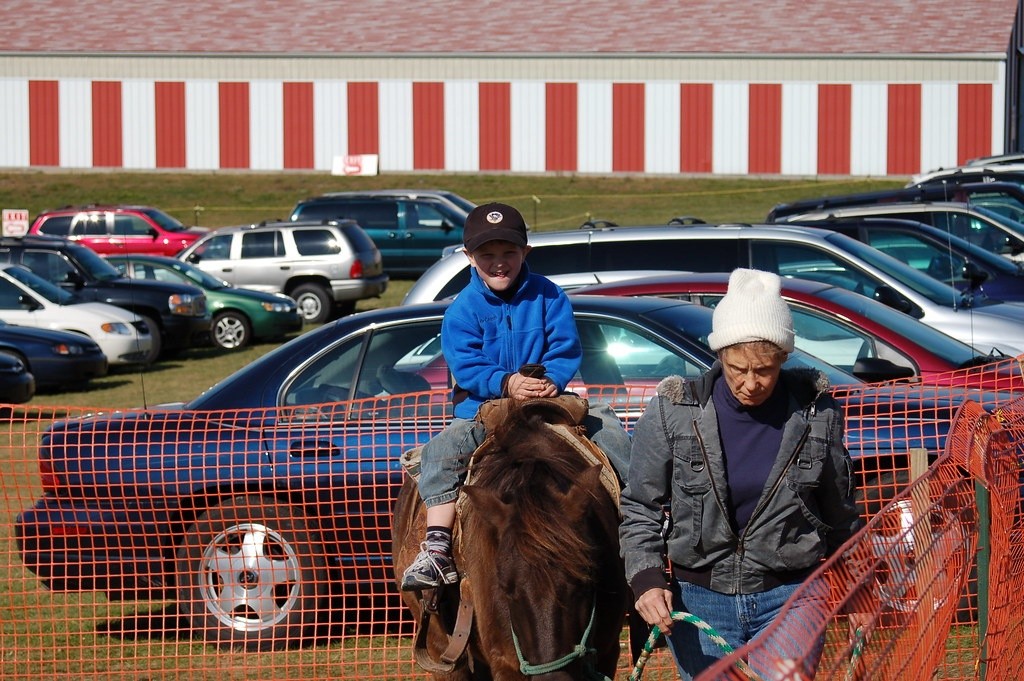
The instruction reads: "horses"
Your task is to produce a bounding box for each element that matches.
[392,401,634,681]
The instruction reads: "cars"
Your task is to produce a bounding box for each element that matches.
[0,319,109,393]
[59,253,302,353]
[0,261,163,370]
[401,151,1024,387]
[0,352,35,404]
[405,270,1024,426]
[15,291,1019,659]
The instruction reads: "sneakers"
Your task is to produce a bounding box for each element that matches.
[401,541,458,591]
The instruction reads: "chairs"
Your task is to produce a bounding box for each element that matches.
[375,365,431,406]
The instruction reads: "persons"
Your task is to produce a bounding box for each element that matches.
[400,203,633,590]
[619,267,877,681]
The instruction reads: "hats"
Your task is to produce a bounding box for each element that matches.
[707,268,795,352]
[462,202,528,253]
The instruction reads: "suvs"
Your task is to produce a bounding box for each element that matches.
[0,235,208,358]
[125,215,390,328]
[286,190,531,280]
[24,203,215,262]
